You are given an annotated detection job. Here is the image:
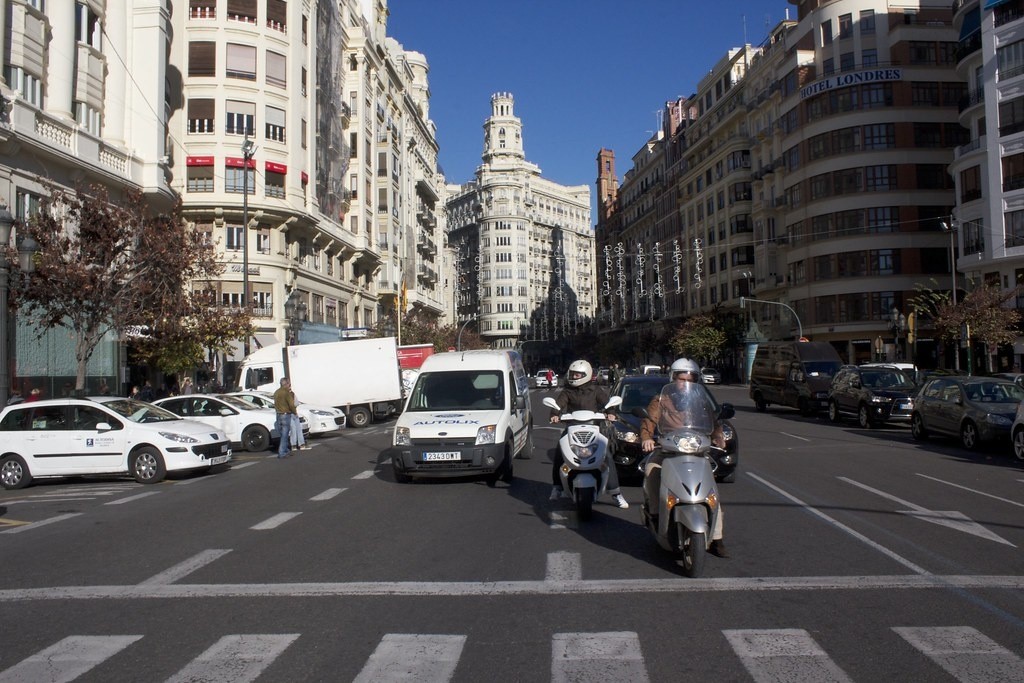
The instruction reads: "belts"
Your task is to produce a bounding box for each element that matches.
[277,412,292,415]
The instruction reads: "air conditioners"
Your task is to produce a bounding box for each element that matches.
[742,161,750,168]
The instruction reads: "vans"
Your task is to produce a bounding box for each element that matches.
[750,340,844,420]
[390,347,533,483]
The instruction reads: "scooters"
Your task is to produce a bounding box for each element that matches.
[541,395,623,519]
[629,380,736,577]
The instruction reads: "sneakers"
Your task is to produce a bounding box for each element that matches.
[611,494,629,509]
[549,487,562,500]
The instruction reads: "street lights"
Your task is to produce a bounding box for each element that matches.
[939,214,961,370]
[741,271,755,339]
[241,139,257,357]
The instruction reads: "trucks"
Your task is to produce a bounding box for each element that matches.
[228,338,404,430]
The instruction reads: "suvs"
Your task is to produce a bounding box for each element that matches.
[828,363,922,430]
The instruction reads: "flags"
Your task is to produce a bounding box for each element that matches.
[393,295,398,314]
[401,277,407,310]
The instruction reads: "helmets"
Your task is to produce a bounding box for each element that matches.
[669,357,701,388]
[566,359,593,389]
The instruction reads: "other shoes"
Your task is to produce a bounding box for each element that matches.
[285,451,295,455]
[290,445,299,450]
[650,512,660,536]
[708,542,730,557]
[279,455,289,459]
[299,444,312,450]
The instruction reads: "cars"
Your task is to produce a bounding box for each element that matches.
[533,369,559,388]
[221,390,347,435]
[1011,399,1024,463]
[127,396,311,453]
[0,394,234,491]
[601,375,741,482]
[565,365,661,388]
[699,366,722,384]
[910,374,1024,451]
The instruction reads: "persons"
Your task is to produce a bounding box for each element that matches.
[173,378,224,395]
[549,359,629,509]
[61,381,76,397]
[274,377,312,458]
[596,364,620,389]
[660,365,670,374]
[129,380,168,403]
[546,368,553,390]
[640,357,730,559]
[96,384,111,396]
[6,387,42,406]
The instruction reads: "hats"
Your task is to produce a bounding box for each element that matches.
[31,389,39,395]
